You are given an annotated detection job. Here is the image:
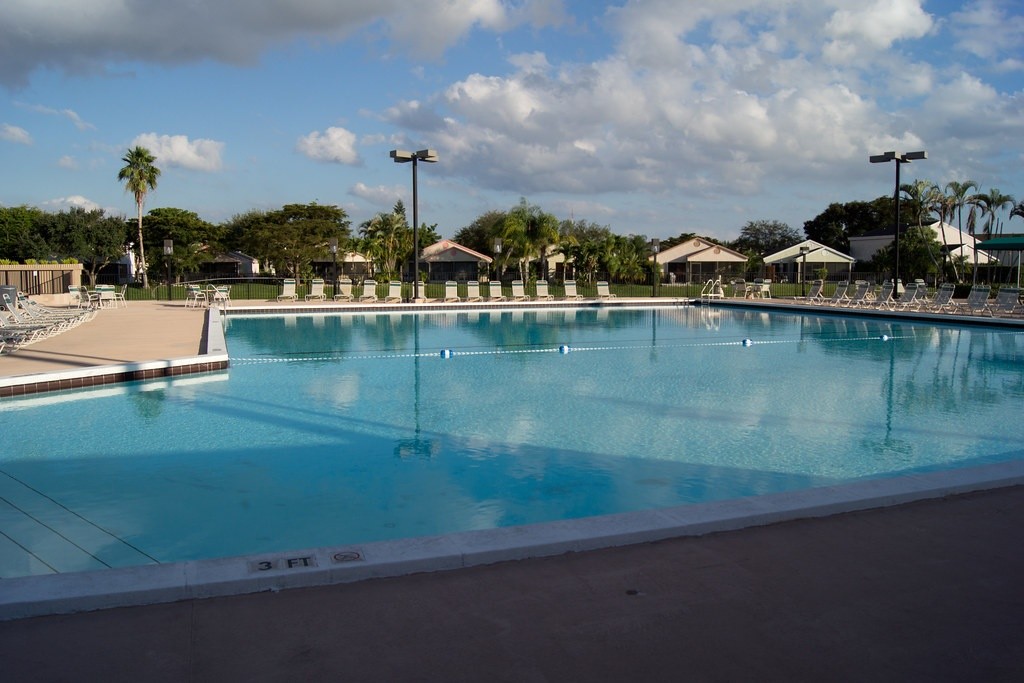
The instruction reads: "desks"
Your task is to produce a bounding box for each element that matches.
[747,283,765,298]
[89,290,100,306]
[200,289,217,306]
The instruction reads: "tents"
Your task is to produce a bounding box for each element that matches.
[973,236,1024,288]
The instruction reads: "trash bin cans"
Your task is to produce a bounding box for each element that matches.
[0,285,18,311]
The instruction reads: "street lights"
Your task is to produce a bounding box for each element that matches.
[390,150,439,303]
[164,240,173,300]
[870,150,927,300]
[800,246,809,297]
[331,245,338,295]
[494,238,502,281]
[650,244,663,297]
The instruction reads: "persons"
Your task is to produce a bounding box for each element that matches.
[670,270,676,284]
[549,272,555,286]
[781,273,788,283]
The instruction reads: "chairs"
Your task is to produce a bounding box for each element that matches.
[536,280,554,301]
[304,279,327,302]
[184,283,233,309]
[411,281,427,305]
[386,281,402,304]
[467,281,484,303]
[442,281,461,303]
[0,285,128,356]
[276,279,299,303]
[489,281,508,302]
[732,278,1024,318]
[596,281,617,300]
[563,280,583,301]
[334,278,354,304]
[512,280,530,301]
[360,280,378,304]
